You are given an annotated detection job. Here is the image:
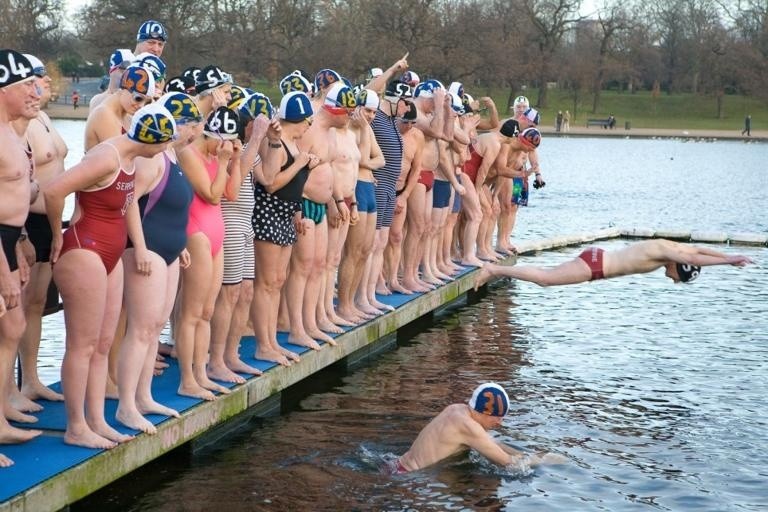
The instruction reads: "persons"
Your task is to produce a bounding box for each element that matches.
[556,110,564,132]
[379,383,547,478]
[562,110,570,132]
[604,114,615,129]
[740,114,752,137]
[0,19,546,468]
[473,236,758,292]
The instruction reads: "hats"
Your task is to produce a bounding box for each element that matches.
[676,260,702,282]
[110,50,273,144]
[137,20,170,44]
[278,71,475,123]
[499,95,540,149]
[470,384,510,417]
[0,50,48,89]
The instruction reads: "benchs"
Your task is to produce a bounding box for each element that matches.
[586,117,614,129]
